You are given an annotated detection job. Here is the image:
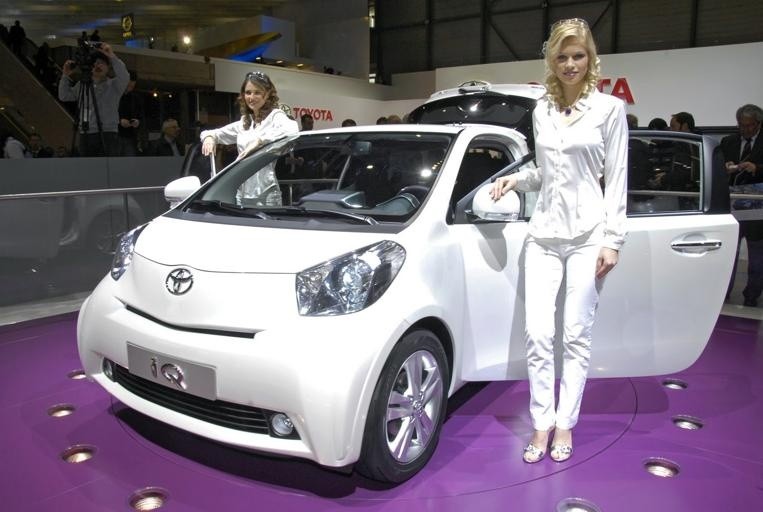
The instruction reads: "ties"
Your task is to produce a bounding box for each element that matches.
[741,138,752,160]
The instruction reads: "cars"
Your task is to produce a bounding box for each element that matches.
[67,81,741,491]
[0,184,148,273]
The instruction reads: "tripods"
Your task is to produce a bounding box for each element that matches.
[71,65,106,157]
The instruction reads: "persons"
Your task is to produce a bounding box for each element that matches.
[0,19,101,88]
[56,42,131,156]
[117,68,140,156]
[489,16,631,463]
[120,118,211,156]
[274,116,424,209]
[628,112,695,215]
[710,103,762,309]
[197,70,298,211]
[0,128,72,159]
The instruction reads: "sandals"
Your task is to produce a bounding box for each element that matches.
[549,427,572,462]
[523,431,548,463]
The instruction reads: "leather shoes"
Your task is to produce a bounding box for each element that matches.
[745,295,756,307]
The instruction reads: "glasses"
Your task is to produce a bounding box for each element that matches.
[246,72,264,79]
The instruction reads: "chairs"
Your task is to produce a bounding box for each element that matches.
[348,149,495,206]
